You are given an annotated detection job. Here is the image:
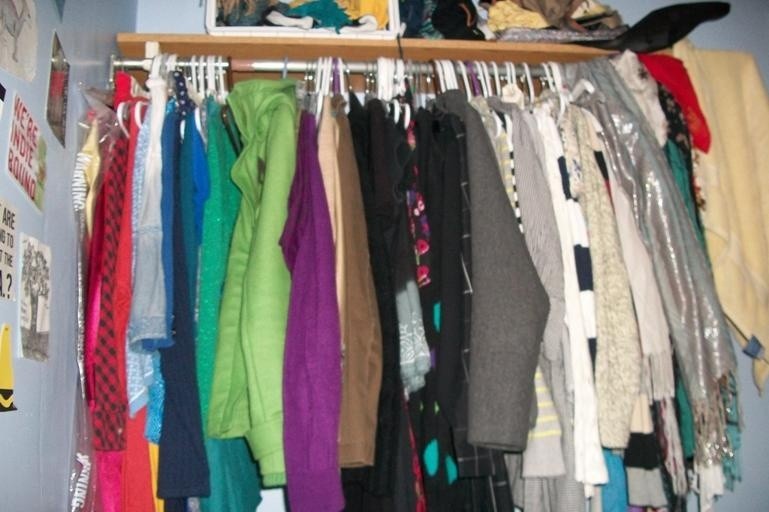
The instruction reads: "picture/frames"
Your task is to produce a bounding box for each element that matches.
[205,0,400,39]
